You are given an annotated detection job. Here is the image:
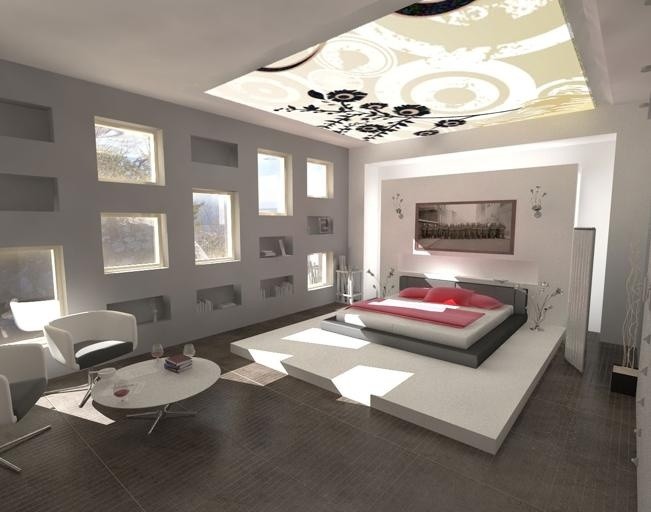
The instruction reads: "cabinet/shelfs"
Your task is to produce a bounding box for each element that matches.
[336,268,361,306]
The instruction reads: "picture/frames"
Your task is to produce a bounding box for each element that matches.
[413,200,517,256]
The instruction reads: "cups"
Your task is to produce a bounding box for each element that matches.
[183,343,196,358]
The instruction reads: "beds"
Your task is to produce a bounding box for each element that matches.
[320,274,529,370]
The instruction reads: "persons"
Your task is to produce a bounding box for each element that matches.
[422,221,507,241]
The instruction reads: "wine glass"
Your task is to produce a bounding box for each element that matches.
[151,342,164,366]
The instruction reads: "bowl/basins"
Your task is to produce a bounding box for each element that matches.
[97,367,115,379]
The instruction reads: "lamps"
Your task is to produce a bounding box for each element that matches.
[391,193,406,218]
[528,184,547,219]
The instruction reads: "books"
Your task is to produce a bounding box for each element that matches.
[260,280,295,301]
[164,354,195,373]
[196,297,239,312]
[260,250,277,259]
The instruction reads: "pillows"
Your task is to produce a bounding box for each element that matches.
[399,287,504,310]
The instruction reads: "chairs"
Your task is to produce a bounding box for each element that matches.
[42,309,139,408]
[0,342,52,473]
[9,297,60,332]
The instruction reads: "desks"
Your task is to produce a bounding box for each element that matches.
[89,353,221,438]
